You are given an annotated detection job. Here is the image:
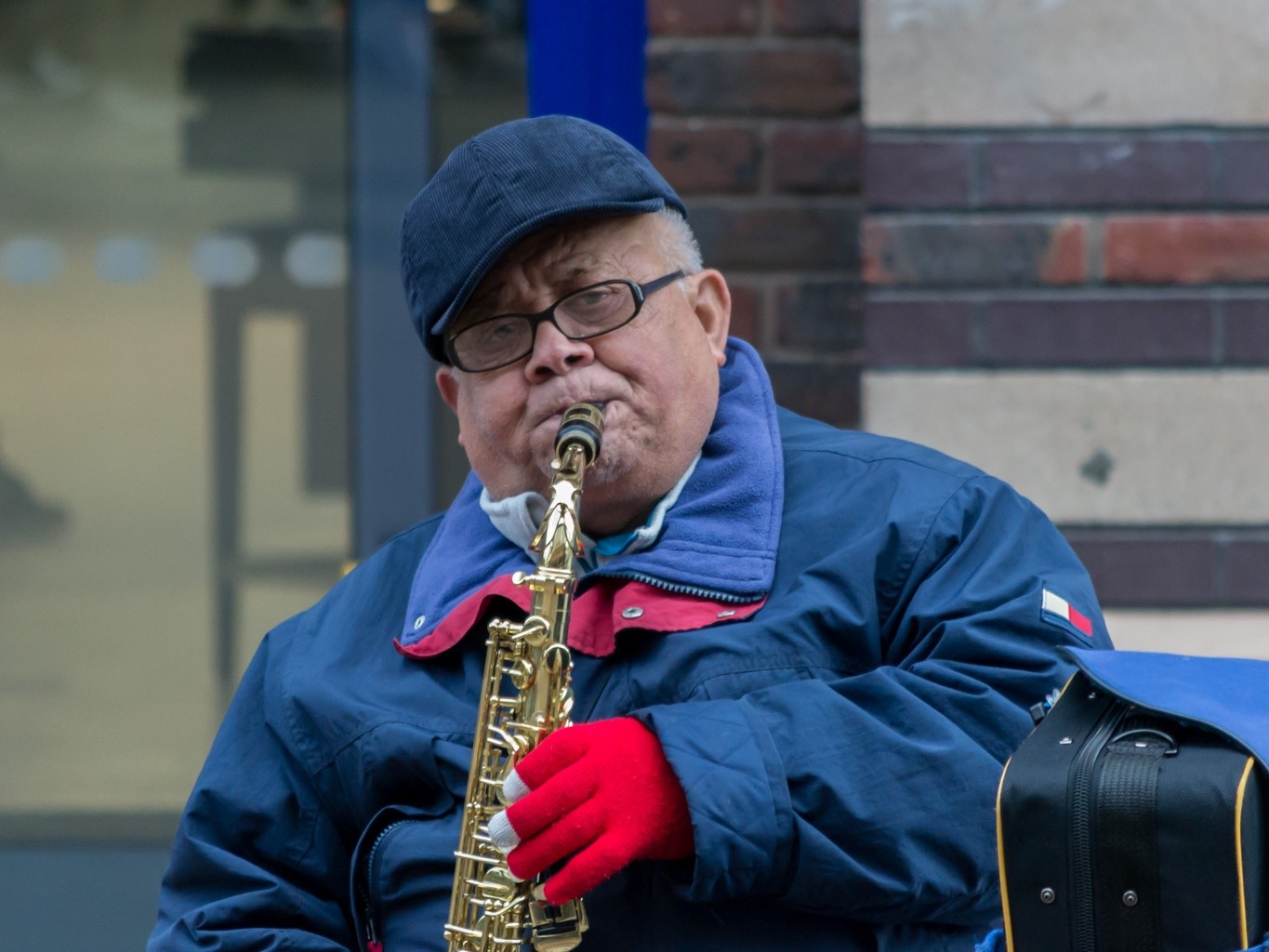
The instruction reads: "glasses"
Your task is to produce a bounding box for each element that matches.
[445,271,689,374]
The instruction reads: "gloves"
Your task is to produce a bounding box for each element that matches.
[488,717,696,904]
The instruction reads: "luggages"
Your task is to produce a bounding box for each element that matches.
[996,644,1269,952]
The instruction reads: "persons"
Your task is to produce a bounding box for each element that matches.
[147,117,1116,952]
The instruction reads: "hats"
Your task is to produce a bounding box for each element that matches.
[398,114,688,364]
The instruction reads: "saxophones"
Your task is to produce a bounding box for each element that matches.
[439,398,611,952]
